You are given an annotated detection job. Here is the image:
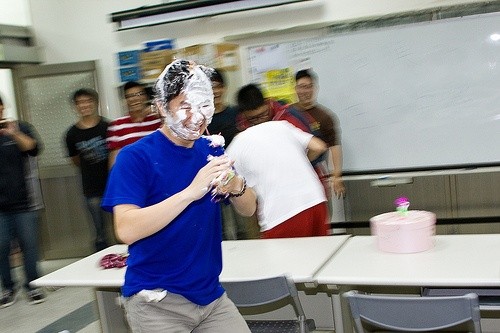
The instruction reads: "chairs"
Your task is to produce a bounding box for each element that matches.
[220,272,316,333]
[342,290,482,333]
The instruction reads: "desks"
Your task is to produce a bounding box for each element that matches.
[29,235,353,333]
[313,234,500,333]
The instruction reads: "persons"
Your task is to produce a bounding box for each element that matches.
[0,99,47,309]
[65,67,347,251]
[99,59,257,333]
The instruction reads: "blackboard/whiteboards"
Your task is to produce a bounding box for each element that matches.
[241,10,500,181]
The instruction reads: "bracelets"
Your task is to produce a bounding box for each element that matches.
[228,177,247,197]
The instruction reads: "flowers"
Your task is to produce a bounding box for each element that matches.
[395,197,410,217]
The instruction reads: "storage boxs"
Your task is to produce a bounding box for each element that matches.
[369,210,436,254]
